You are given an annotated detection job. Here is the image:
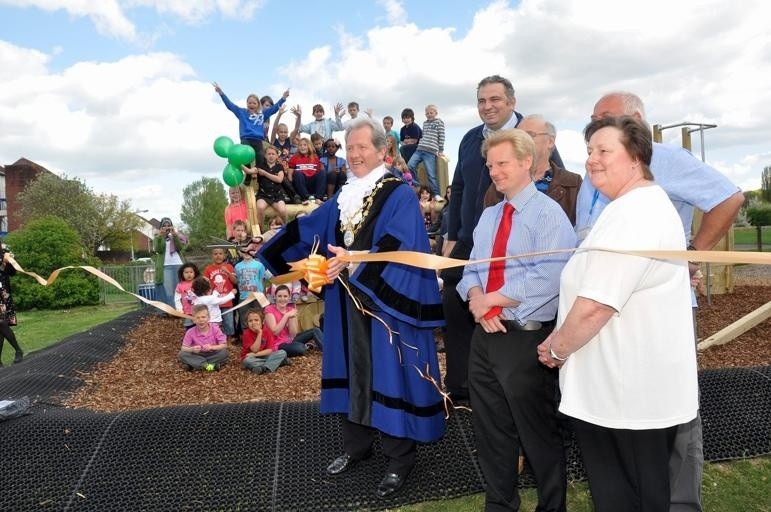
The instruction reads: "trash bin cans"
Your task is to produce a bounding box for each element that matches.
[139,284,155,308]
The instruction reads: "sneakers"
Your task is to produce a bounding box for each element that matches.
[204,361,221,372]
[251,357,293,374]
[301,198,324,205]
[434,194,445,202]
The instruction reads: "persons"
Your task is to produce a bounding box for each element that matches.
[337,100,453,257]
[441,77,584,510]
[157,215,188,318]
[0,243,24,367]
[212,79,344,269]
[249,121,446,500]
[574,93,744,512]
[174,245,324,373]
[535,115,699,512]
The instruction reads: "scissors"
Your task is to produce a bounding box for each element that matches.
[205,236,264,257]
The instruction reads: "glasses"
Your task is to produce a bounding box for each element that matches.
[526,129,550,139]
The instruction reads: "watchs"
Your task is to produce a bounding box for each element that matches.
[550,350,568,362]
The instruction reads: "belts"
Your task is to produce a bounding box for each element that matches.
[498,318,543,332]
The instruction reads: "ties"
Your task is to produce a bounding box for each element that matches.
[482,202,516,321]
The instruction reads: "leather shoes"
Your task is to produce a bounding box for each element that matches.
[327,446,373,476]
[376,461,417,501]
